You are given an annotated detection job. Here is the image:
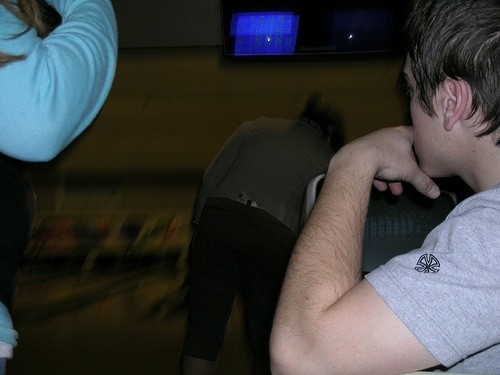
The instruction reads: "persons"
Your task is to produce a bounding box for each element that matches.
[179,100,346,375]
[0,0,119,374]
[270,0,500,375]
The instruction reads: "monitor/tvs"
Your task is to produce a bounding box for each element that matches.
[220,0,413,64]
